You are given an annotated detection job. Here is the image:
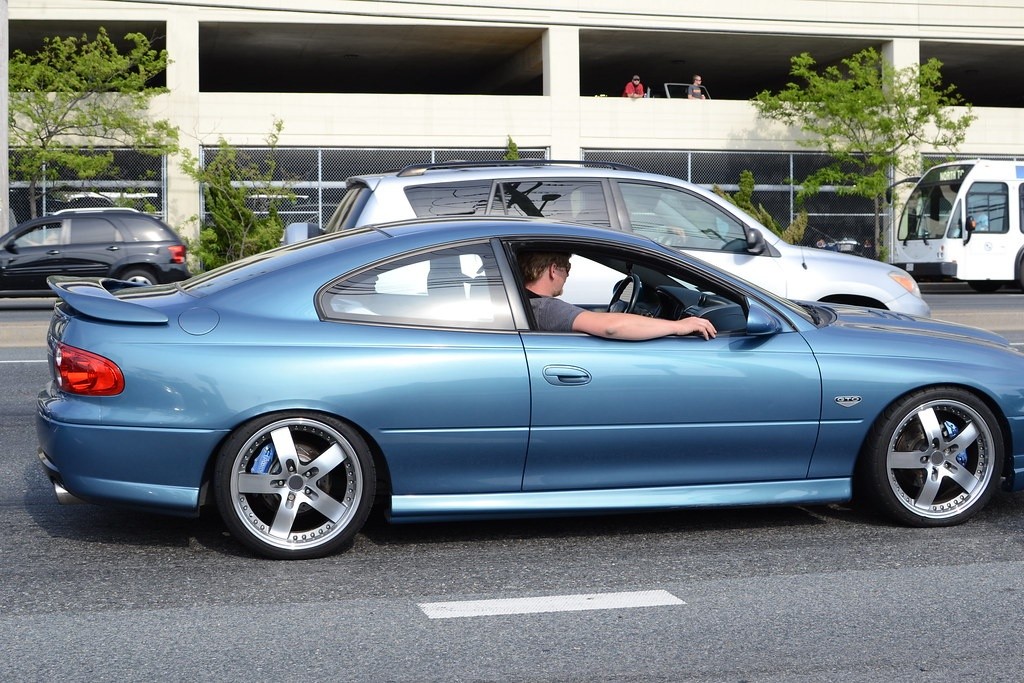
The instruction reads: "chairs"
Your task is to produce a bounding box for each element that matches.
[469,276,496,324]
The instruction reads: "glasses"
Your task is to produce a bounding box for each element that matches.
[560,263,571,274]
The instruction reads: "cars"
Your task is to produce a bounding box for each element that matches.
[35,221,1024,561]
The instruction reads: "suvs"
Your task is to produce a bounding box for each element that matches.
[281,158,931,315]
[0,207,191,308]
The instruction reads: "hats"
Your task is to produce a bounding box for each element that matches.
[633,75,639,79]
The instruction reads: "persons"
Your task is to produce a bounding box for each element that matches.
[23,231,59,246]
[973,209,988,230]
[687,75,705,99]
[624,75,643,97]
[516,240,717,341]
[629,186,686,246]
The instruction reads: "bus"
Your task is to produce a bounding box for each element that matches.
[885,160,1024,293]
